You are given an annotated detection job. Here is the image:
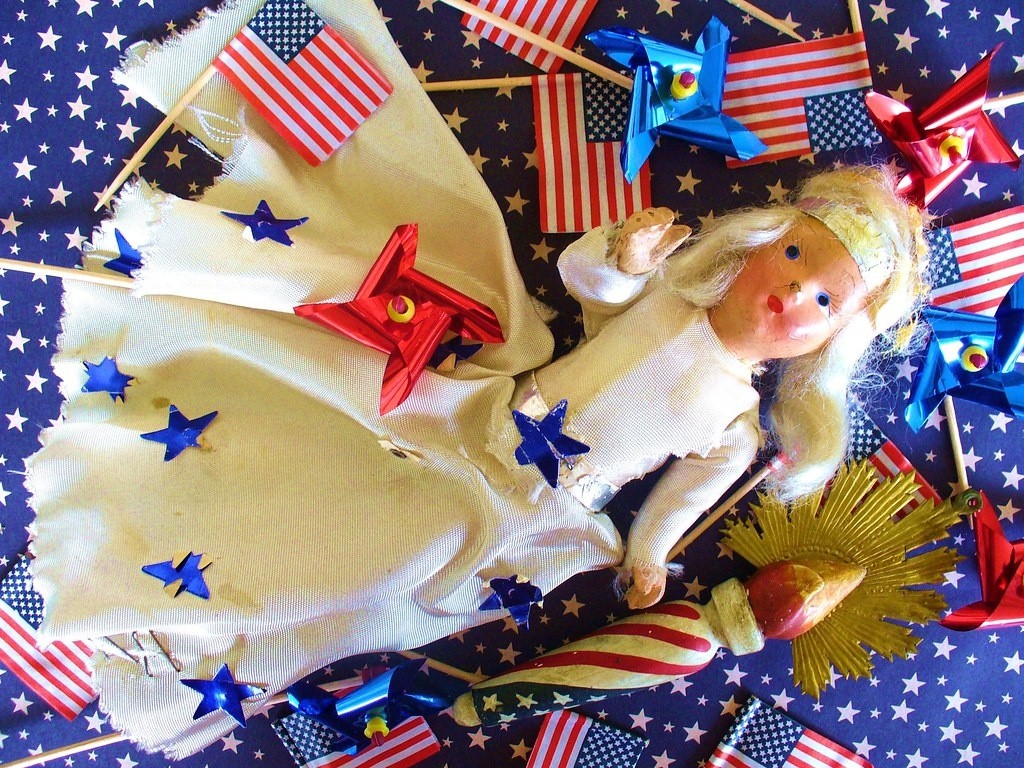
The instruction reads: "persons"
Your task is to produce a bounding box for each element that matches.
[28,0,931,749]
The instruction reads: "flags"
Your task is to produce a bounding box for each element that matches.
[0,0,1024,768]
